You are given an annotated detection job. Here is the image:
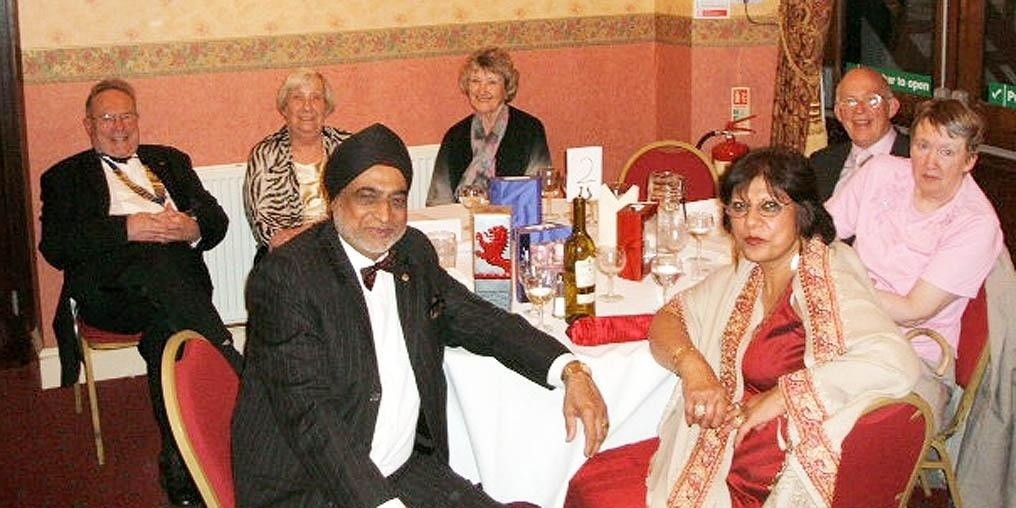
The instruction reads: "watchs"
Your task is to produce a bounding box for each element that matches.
[560,359,592,381]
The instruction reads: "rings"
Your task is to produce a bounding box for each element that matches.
[692,401,707,418]
[732,400,744,425]
[601,423,609,428]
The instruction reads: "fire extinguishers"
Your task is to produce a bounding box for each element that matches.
[695,115,756,179]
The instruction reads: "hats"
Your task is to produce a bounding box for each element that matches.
[323,122,412,200]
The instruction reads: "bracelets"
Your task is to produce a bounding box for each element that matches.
[668,344,699,375]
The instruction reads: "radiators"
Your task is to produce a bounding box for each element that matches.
[185,144,442,325]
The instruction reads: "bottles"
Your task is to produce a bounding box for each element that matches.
[564,197,599,324]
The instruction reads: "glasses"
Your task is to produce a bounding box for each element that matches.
[834,91,894,111]
[722,195,797,216]
[89,111,142,123]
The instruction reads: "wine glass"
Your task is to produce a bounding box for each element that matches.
[539,168,561,219]
[526,263,558,334]
[600,213,718,309]
[461,184,487,241]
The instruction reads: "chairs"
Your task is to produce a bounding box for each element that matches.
[618,143,721,204]
[71,305,143,465]
[823,391,933,508]
[912,245,1012,508]
[160,330,243,508]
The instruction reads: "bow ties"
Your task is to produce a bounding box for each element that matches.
[360,252,398,292]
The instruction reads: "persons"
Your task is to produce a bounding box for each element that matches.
[426,44,554,208]
[231,123,610,508]
[37,79,245,508]
[809,68,1006,401]
[563,146,921,508]
[242,67,353,265]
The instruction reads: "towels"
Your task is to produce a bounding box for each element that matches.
[596,183,641,245]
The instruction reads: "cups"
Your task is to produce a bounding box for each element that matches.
[426,230,459,269]
[649,172,687,216]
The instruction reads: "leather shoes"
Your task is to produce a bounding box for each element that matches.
[158,454,204,508]
